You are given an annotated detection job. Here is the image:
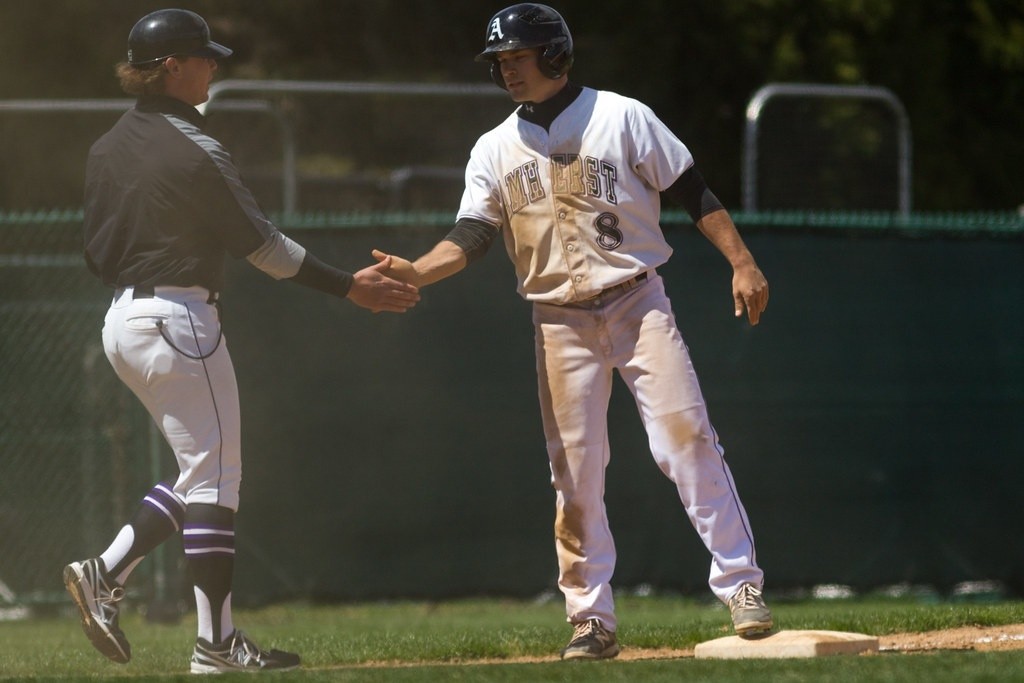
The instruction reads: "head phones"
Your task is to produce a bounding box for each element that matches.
[489,43,573,92]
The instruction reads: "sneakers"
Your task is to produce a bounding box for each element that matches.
[190,629,300,674]
[561,618,620,659]
[728,583,773,638]
[62,556,131,663]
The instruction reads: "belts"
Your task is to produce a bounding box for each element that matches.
[585,271,647,301]
[133,286,215,304]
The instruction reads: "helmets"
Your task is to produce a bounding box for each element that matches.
[474,3,574,90]
[128,9,233,65]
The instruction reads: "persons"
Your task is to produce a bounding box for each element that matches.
[62,9,420,674]
[373,4,774,661]
